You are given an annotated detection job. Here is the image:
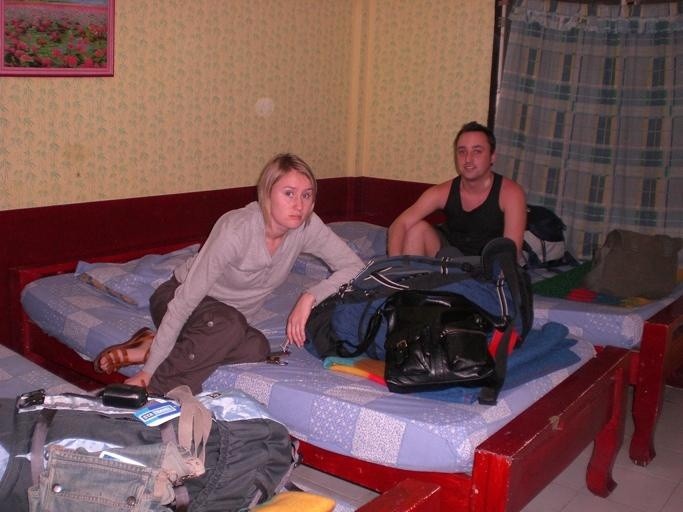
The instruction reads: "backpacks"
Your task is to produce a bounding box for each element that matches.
[1,388,302,511]
[302,237,536,362]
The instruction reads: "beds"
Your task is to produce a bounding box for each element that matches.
[19,236,630,512]
[288,221,683,467]
[3,341,440,512]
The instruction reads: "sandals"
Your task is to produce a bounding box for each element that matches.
[92,326,156,376]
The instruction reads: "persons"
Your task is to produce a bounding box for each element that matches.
[88,150,367,402]
[386,119,526,265]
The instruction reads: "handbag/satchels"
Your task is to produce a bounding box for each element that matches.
[27,383,213,511]
[582,228,682,301]
[336,288,514,406]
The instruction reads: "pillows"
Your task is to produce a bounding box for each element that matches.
[75,244,202,308]
[291,222,390,280]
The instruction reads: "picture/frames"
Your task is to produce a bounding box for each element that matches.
[0,0,116,77]
